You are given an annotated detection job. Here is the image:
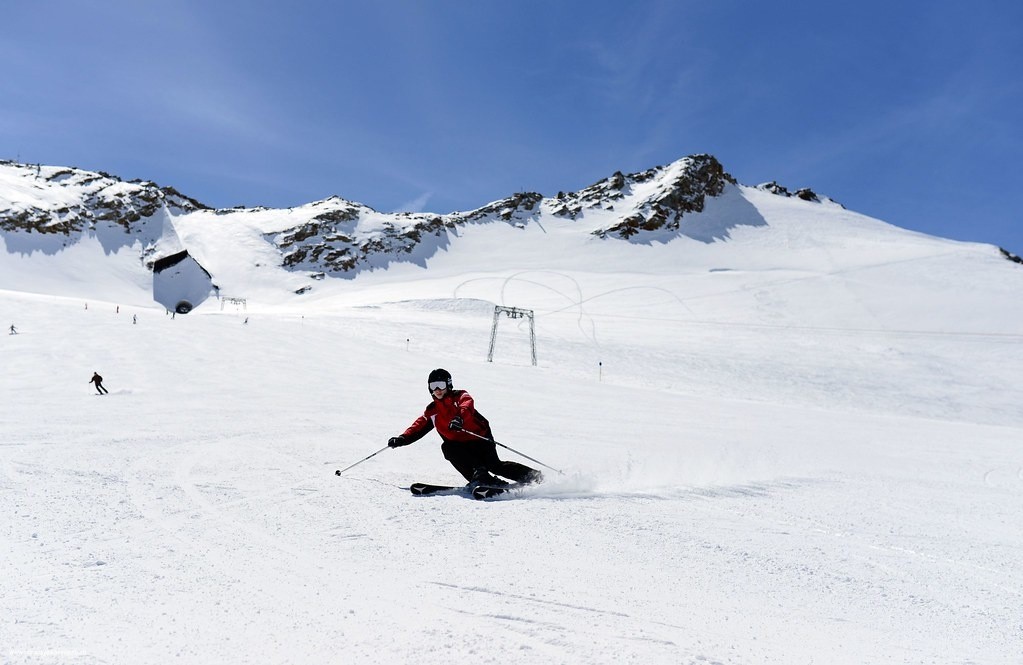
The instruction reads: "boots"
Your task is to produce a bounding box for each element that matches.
[517,468,543,485]
[465,467,509,494]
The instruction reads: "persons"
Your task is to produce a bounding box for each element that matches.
[88,371,108,395]
[116,306,120,313]
[166,308,169,316]
[9,324,18,333]
[85,301,88,310]
[170,311,177,319]
[244,317,248,322]
[386,369,541,494]
[133,315,137,323]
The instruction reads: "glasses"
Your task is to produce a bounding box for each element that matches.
[429,379,452,391]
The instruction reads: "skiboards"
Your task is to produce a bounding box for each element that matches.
[410,469,538,500]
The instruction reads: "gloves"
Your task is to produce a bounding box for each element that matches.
[388,437,403,449]
[448,415,463,432]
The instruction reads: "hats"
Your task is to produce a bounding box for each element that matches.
[428,369,453,397]
